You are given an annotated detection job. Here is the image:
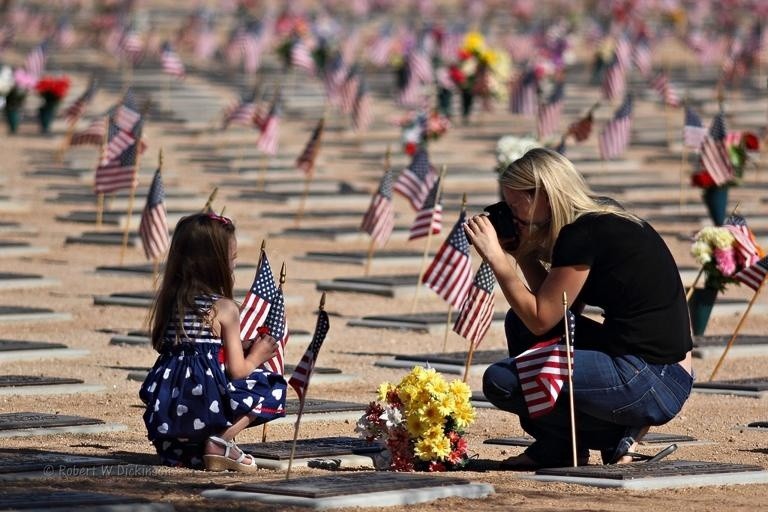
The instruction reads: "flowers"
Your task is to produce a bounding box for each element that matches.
[448,32,512,102]
[355,365,478,475]
[692,131,761,188]
[691,224,742,295]
[0,66,71,103]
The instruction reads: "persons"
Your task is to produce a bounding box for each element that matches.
[138,211,287,473]
[462,144,696,474]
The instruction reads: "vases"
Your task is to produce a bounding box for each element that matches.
[704,189,728,225]
[686,288,717,335]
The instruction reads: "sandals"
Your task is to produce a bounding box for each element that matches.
[202,435,258,474]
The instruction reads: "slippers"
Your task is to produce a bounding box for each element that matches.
[622,444,678,464]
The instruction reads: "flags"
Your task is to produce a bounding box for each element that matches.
[287,307,331,402]
[718,212,759,267]
[0,0,768,250]
[729,253,768,292]
[419,207,476,311]
[237,251,276,341]
[137,168,169,263]
[252,284,290,381]
[450,260,496,350]
[513,308,577,420]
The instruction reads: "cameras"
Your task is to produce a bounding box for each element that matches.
[464,199,523,251]
